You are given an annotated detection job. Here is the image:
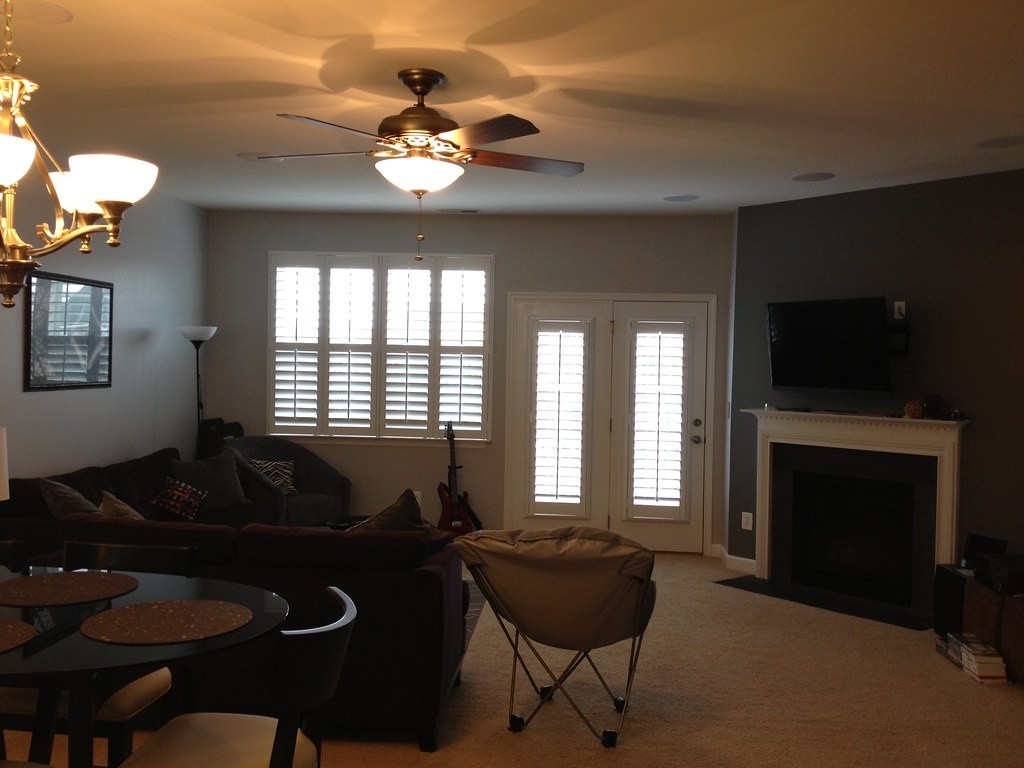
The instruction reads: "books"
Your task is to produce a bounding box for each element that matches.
[935,631,1012,686]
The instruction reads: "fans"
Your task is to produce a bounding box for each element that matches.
[258,67,584,179]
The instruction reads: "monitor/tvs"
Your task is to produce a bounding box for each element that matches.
[767,297,892,392]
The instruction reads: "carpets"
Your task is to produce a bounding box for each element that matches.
[714,576,937,631]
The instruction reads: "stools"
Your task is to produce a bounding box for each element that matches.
[935,564,1024,685]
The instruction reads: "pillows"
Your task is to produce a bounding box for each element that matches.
[250,458,299,497]
[229,447,284,498]
[101,490,146,521]
[38,478,103,518]
[346,489,456,552]
[150,476,209,520]
[169,450,252,514]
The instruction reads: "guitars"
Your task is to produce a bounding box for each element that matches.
[437,421,484,534]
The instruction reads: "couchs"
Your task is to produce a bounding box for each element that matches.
[0,435,470,752]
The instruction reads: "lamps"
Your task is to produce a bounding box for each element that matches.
[376,158,465,261]
[0,0,160,308]
[178,326,218,455]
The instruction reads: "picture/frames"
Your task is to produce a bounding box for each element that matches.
[22,270,114,392]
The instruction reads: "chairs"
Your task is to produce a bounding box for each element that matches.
[0,542,199,768]
[117,585,357,768]
[453,526,657,749]
